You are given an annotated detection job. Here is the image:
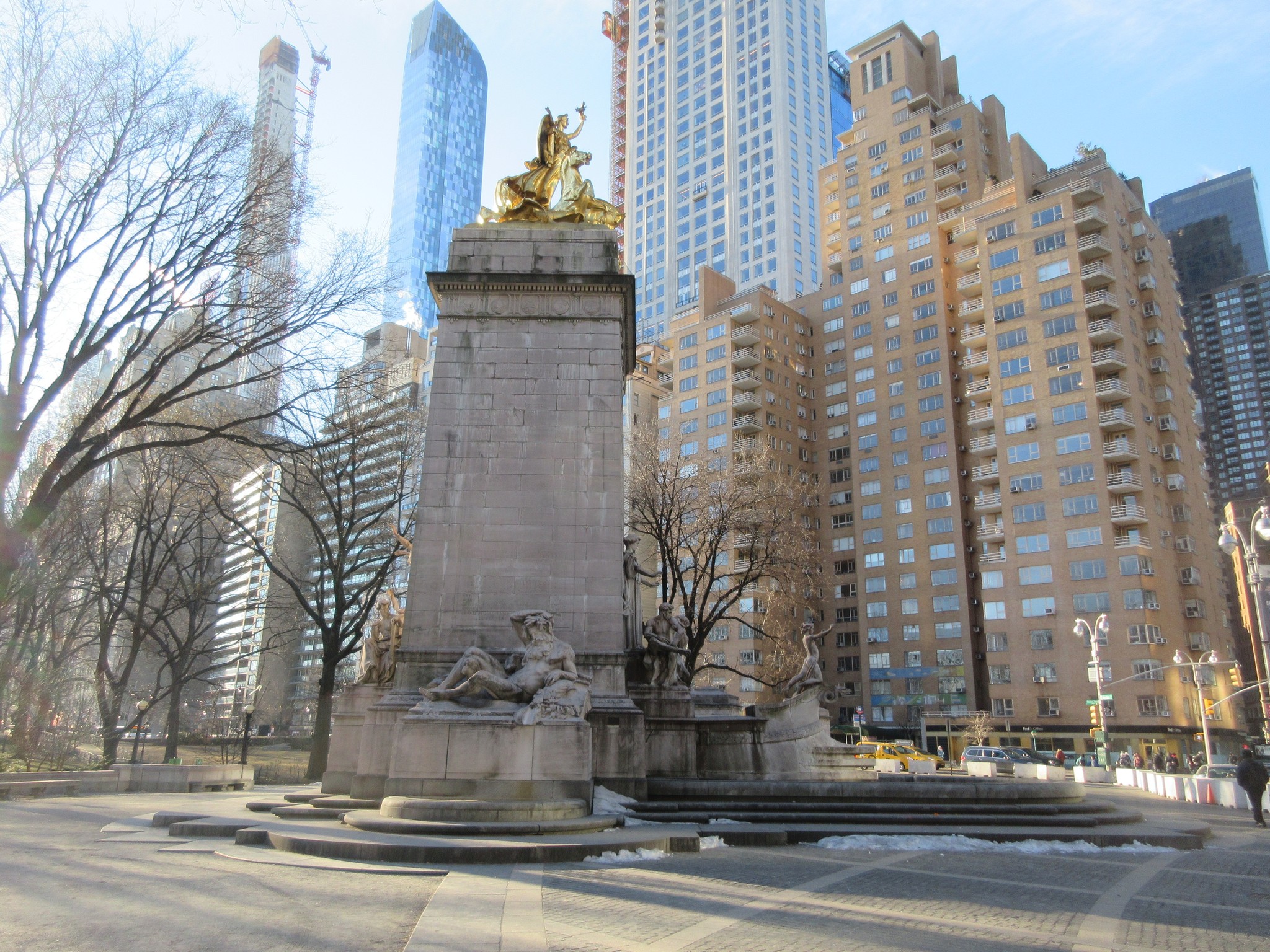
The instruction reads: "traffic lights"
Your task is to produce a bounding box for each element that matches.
[306,704,314,714]
[1194,734,1202,742]
[1203,699,1214,715]
[1090,704,1099,724]
[1228,668,1244,687]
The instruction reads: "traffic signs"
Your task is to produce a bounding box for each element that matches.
[1103,706,1111,713]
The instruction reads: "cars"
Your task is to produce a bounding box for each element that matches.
[1191,765,1241,780]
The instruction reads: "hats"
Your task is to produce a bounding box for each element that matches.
[1242,749,1252,758]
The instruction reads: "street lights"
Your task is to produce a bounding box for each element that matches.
[130,697,149,763]
[1217,497,1270,692]
[1073,612,1113,774]
[238,702,255,764]
[1172,649,1218,771]
[1030,730,1037,751]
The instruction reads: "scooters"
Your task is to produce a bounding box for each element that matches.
[1164,760,1176,774]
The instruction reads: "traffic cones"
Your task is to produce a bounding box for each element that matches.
[1206,782,1218,805]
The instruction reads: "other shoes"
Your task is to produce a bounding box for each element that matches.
[1257,823,1266,827]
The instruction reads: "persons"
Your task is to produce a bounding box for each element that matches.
[1229,753,1238,765]
[419,609,578,702]
[937,746,944,758]
[1186,751,1207,774]
[1132,751,1144,769]
[783,622,834,698]
[622,533,663,649]
[1087,755,1098,767]
[1151,751,1179,774]
[1236,749,1269,827]
[358,588,405,683]
[501,112,585,210]
[643,602,691,691]
[1116,749,1131,768]
[1076,754,1087,766]
[1056,748,1065,768]
[389,523,413,565]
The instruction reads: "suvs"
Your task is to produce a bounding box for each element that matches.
[854,741,945,772]
[960,745,1062,774]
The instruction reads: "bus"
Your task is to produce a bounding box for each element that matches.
[96,725,152,738]
